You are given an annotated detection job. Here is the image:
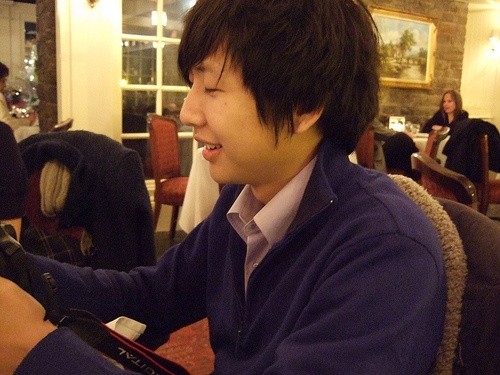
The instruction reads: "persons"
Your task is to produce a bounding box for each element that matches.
[372,117,399,174]
[0,61,36,131]
[421,88,480,182]
[0,0,450,375]
[0,121,31,244]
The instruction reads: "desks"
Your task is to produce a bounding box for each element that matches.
[412,134,449,167]
[179,147,219,235]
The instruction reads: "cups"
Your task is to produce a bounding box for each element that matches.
[410,124,420,134]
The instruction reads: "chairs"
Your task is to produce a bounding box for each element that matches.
[147,114,191,238]
[15,130,152,273]
[411,124,500,374]
[356,130,374,169]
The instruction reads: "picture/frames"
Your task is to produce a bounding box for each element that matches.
[367,4,438,89]
